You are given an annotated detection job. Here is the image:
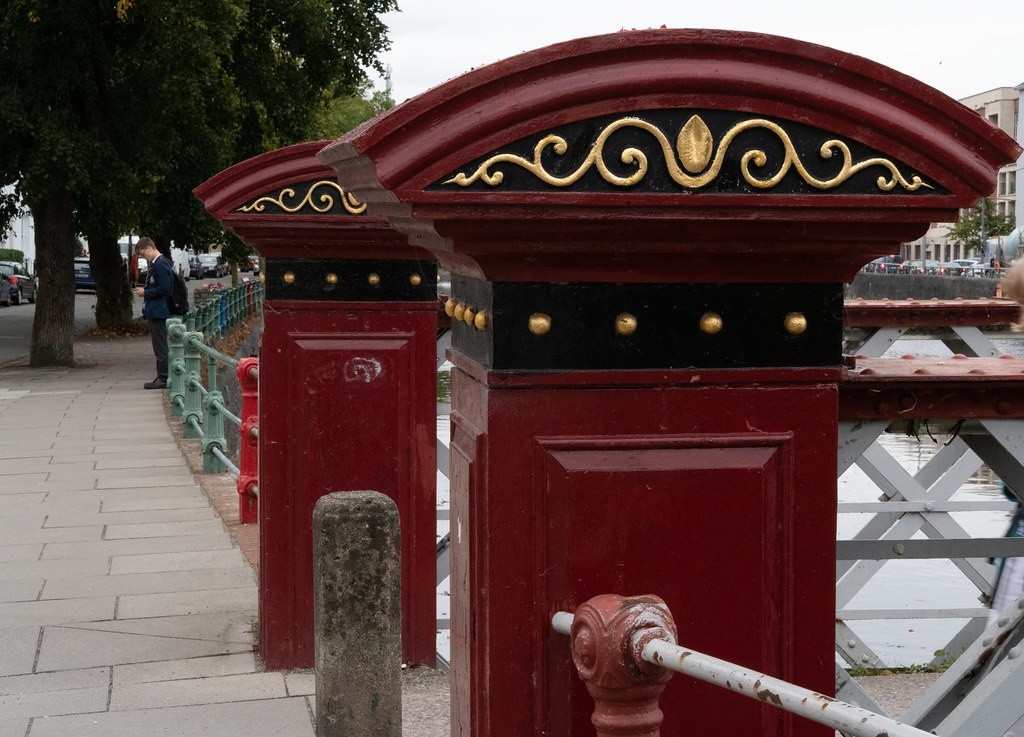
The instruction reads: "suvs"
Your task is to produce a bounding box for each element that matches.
[74,257,97,292]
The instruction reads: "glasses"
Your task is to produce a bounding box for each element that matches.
[140,248,147,258]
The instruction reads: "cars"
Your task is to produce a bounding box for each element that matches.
[0,260,38,307]
[865,255,994,279]
[122,252,260,287]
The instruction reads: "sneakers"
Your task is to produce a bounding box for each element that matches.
[144,378,167,389]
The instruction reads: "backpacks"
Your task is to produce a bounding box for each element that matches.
[152,258,189,316]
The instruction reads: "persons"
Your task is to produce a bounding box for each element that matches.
[133,237,171,389]
